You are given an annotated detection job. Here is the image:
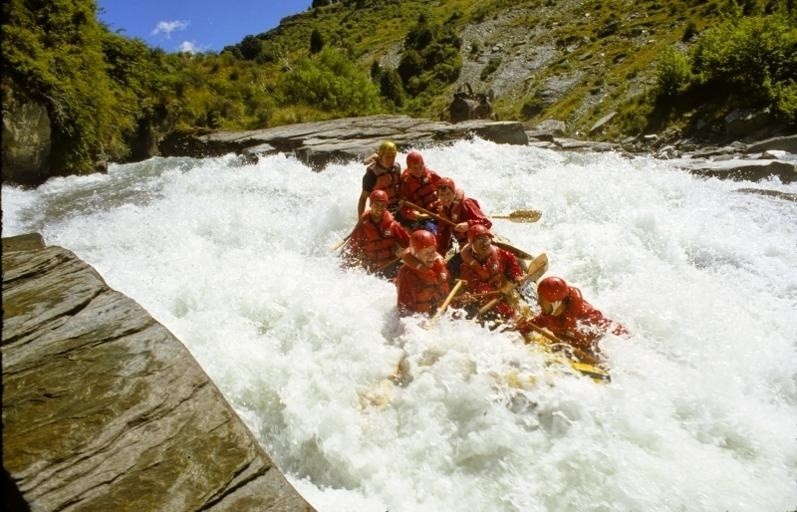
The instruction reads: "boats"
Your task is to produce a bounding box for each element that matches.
[340,223,611,397]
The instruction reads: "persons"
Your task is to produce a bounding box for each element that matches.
[515,275,632,354]
[337,188,413,285]
[398,150,442,235]
[433,175,493,257]
[394,228,469,334]
[445,224,525,320]
[357,140,402,221]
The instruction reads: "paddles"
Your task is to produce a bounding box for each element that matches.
[399,197,534,260]
[492,209,542,223]
[480,253,548,312]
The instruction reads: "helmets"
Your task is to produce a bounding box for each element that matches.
[467,225,494,243]
[410,229,437,255]
[537,276,570,303]
[370,190,388,205]
[436,176,455,193]
[406,151,423,166]
[379,142,396,157]
